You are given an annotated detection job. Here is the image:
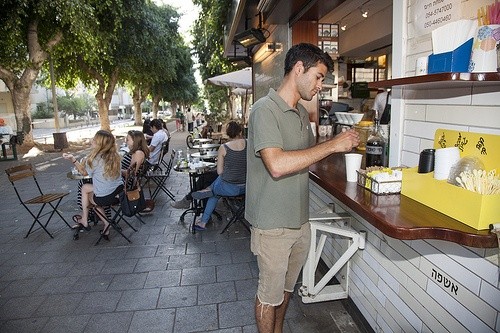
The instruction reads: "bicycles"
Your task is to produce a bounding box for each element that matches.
[186,127,202,148]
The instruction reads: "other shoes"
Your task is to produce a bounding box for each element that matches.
[193,224,207,231]
[191,187,212,201]
[169,196,191,209]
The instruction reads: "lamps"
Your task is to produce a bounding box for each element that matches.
[252,42,275,65]
[360,8,369,19]
[233,0,269,49]
[341,23,348,31]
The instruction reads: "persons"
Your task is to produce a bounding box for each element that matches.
[175,108,205,132]
[143,119,170,166]
[171,120,247,231]
[245,43,361,333]
[78,130,150,220]
[63,130,124,240]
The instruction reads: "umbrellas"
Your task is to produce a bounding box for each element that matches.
[205,67,252,95]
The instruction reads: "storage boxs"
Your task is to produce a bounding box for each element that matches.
[401,130,500,230]
[428,36,474,74]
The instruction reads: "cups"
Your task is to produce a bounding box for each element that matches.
[199,142,202,147]
[335,111,364,133]
[345,154,364,183]
[309,122,317,137]
[318,125,333,136]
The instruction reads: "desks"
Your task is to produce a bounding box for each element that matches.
[194,143,222,153]
[68,171,100,227]
[192,138,212,144]
[174,161,223,222]
[190,151,218,162]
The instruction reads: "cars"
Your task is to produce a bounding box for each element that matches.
[142,111,171,119]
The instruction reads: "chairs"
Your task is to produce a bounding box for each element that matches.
[5,162,71,238]
[95,136,253,246]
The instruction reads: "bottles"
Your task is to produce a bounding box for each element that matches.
[417,149,436,174]
[433,147,461,181]
[366,118,387,168]
[186,147,191,162]
[415,57,428,76]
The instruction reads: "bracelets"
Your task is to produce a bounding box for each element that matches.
[73,160,77,163]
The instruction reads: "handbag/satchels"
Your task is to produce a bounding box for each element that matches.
[118,174,147,217]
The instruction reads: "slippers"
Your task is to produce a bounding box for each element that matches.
[73,215,91,232]
[99,230,110,241]
